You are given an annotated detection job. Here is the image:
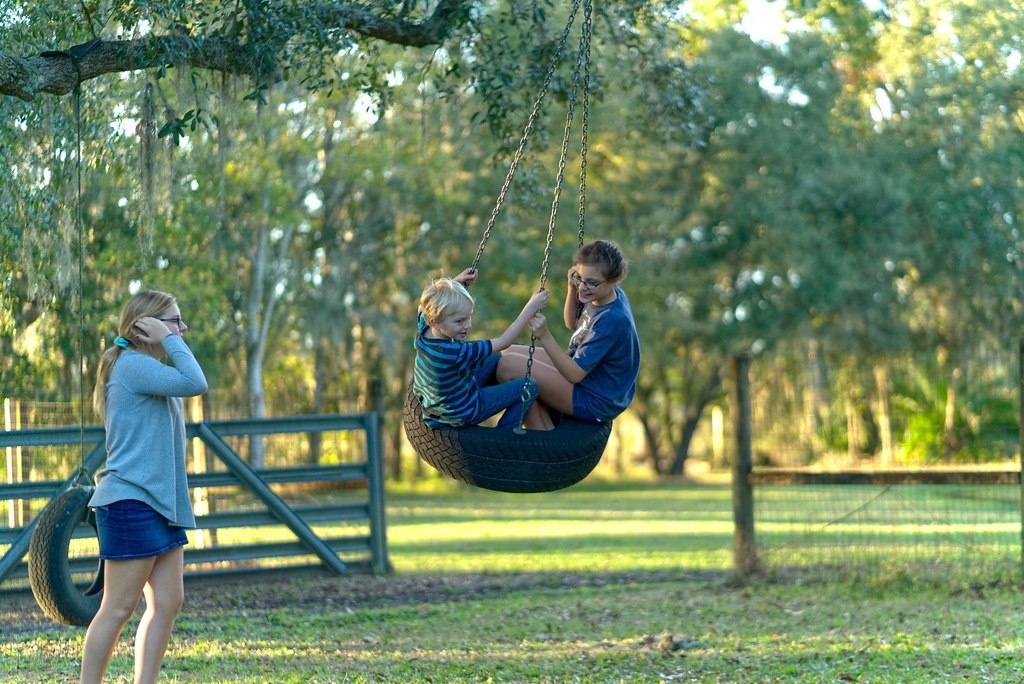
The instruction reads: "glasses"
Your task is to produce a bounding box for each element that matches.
[571,269,607,290]
[160,315,182,328]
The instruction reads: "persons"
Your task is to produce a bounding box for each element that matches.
[496,240,640,431]
[413,268,551,430]
[80,290,208,684]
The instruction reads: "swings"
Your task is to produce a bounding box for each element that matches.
[28,82,104,626]
[404,1,614,493]
